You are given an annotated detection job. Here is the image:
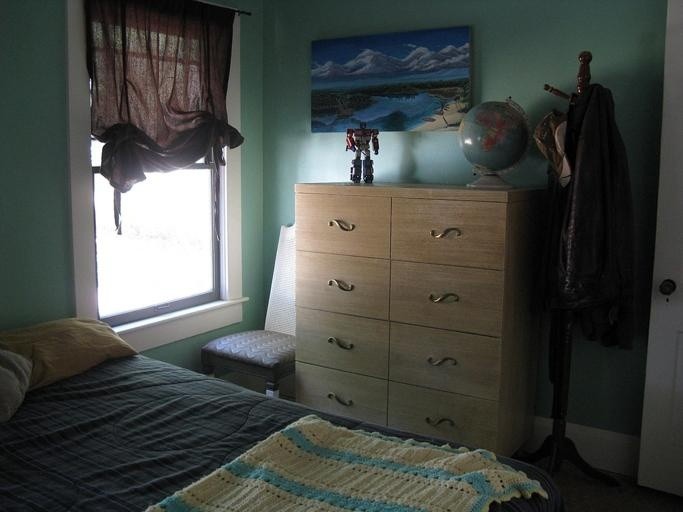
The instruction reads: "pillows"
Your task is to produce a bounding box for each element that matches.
[0,349,33,423]
[0,317,138,393]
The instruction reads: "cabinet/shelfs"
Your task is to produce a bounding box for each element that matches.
[295,182,547,461]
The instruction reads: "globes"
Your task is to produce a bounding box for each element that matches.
[458,96,532,189]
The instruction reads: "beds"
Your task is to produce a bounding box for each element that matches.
[0,319,567,511]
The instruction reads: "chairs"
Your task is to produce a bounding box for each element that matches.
[201,221,295,401]
